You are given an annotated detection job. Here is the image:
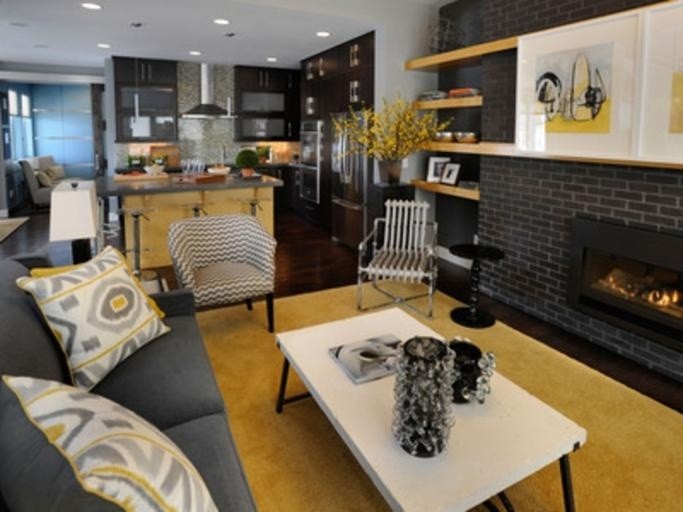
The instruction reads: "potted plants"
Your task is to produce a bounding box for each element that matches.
[234,149,259,178]
[329,92,455,184]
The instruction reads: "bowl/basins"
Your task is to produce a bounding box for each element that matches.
[455,133,477,141]
[144,166,163,175]
[431,132,450,140]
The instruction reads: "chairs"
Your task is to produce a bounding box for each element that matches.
[166,213,278,333]
[356,199,439,319]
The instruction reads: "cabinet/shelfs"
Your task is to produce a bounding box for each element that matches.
[299,29,375,247]
[233,65,300,142]
[112,56,179,146]
[405,35,519,199]
[366,180,415,266]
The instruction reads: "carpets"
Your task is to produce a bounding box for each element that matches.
[194,276,683,512]
[0,216,31,243]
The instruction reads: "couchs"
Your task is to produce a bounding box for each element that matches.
[18,154,81,208]
[0,249,256,512]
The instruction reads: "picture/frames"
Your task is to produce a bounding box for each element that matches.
[425,156,453,183]
[439,161,461,186]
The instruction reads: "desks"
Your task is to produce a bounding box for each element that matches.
[448,242,504,328]
[96,171,284,270]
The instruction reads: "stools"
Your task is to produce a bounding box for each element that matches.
[179,201,215,217]
[115,206,155,281]
[240,197,271,217]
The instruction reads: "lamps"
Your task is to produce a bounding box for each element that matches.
[48,179,101,265]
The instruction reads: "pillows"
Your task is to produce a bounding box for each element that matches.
[30,248,165,319]
[1,373,219,512]
[47,164,66,179]
[15,244,171,391]
[38,171,52,187]
